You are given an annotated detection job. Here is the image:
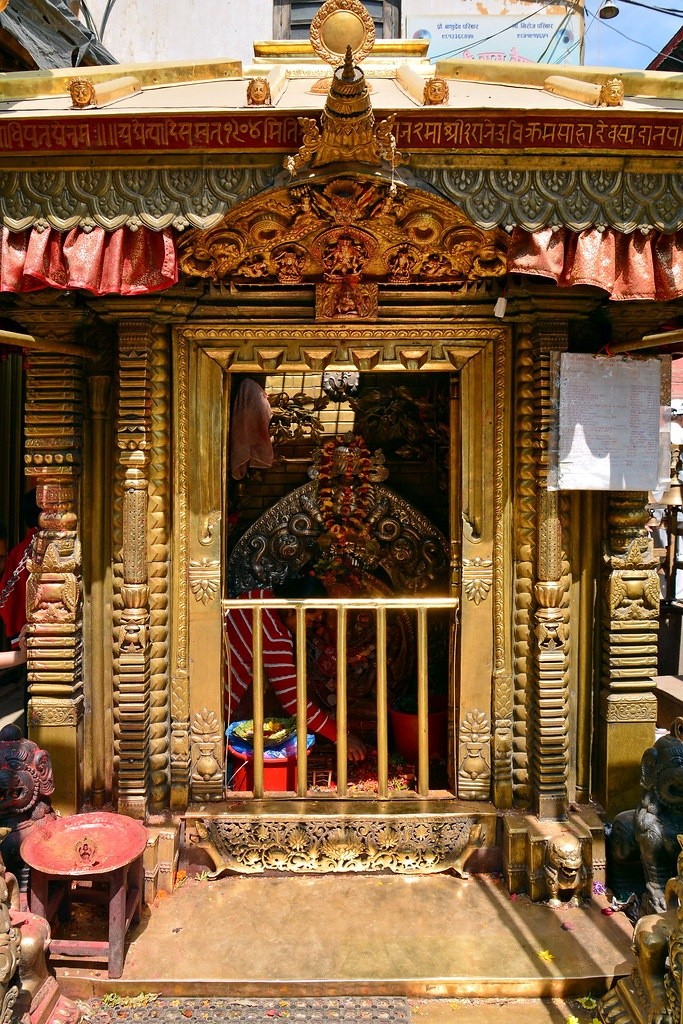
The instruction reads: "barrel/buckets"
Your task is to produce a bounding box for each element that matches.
[387,695,448,759]
[228,743,313,792]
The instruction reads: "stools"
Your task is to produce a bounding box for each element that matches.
[28,846,148,982]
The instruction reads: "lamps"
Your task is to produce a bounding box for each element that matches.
[599,0,620,19]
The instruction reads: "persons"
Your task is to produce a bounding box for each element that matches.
[0,487,40,729]
[221,578,367,764]
